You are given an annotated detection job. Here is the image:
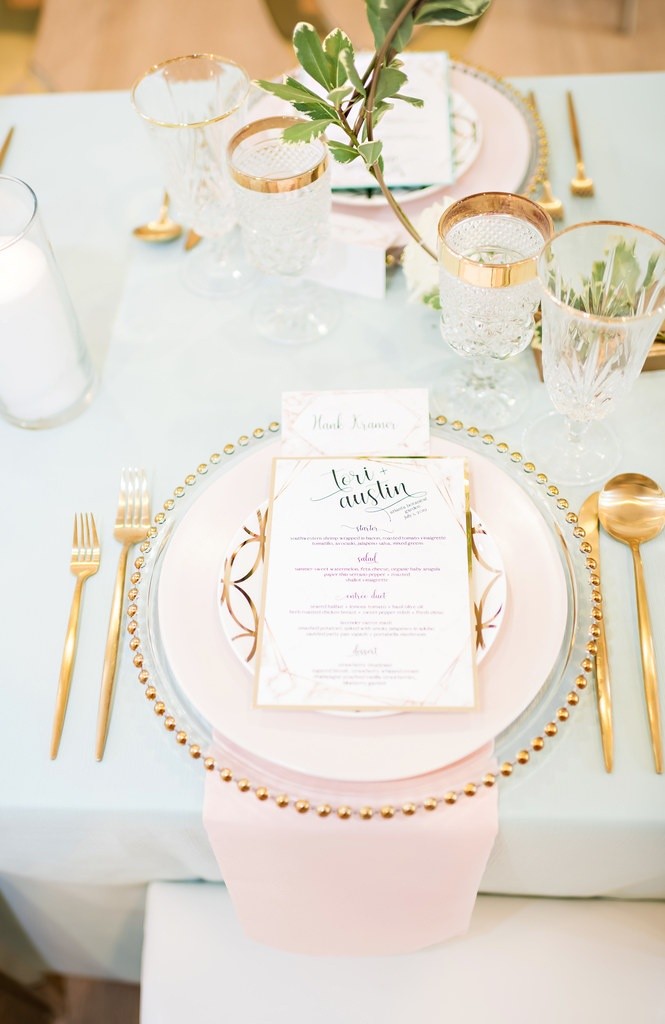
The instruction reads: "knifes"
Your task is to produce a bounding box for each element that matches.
[576,492,614,772]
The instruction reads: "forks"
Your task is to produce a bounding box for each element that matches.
[566,91,594,198]
[49,511,101,761]
[535,181,564,223]
[95,466,152,762]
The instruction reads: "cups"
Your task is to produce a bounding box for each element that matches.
[0,173,93,431]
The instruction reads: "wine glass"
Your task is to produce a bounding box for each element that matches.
[519,220,665,486]
[434,190,554,431]
[131,53,253,296]
[226,114,343,345]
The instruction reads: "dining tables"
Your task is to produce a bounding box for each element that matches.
[1,70,665,986]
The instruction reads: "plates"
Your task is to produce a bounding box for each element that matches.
[127,409,603,821]
[211,55,550,269]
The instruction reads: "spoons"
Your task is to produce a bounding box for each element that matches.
[597,472,665,776]
[133,192,182,242]
